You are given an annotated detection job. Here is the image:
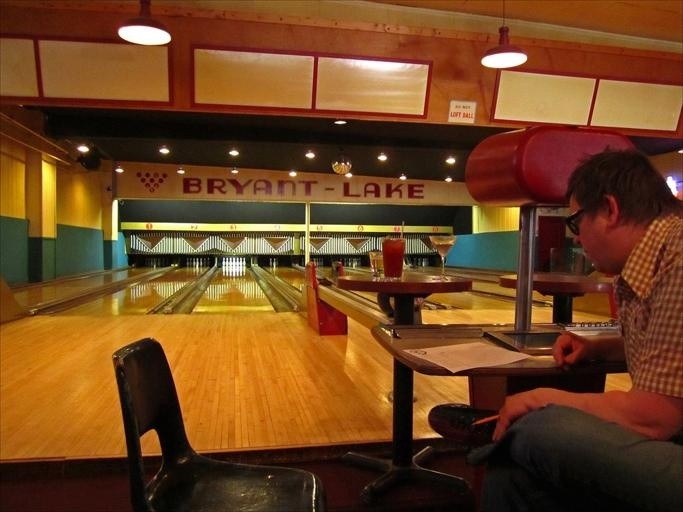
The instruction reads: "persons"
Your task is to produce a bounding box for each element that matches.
[426,146,681,509]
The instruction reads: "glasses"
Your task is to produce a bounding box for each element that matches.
[565,196,604,236]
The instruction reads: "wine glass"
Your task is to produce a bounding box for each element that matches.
[429,235,456,282]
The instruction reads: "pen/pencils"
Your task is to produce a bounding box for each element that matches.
[471,415,501,426]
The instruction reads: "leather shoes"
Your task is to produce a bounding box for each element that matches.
[427,402,498,446]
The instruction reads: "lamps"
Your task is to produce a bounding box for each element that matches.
[480,0,528,69]
[116,0,172,46]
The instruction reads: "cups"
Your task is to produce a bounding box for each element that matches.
[369,237,406,280]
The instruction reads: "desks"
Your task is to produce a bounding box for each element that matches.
[370,323,630,511]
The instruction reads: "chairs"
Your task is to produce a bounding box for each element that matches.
[111,336,328,512]
